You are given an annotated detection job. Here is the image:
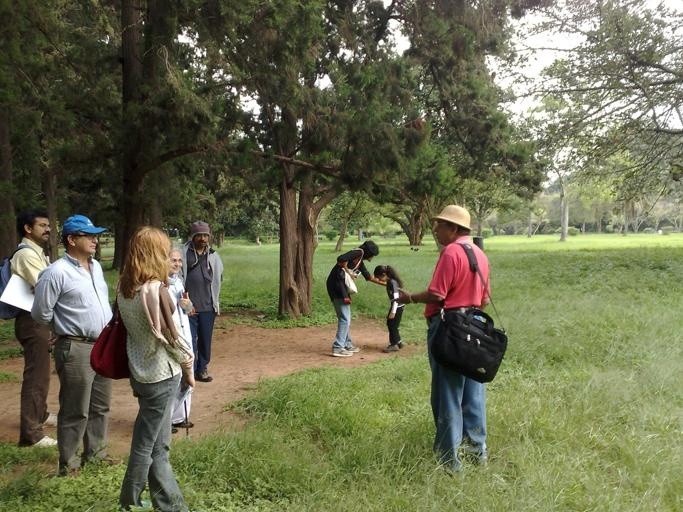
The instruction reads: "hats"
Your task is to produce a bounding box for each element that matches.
[430,204,474,231]
[191,220,212,272]
[61,215,107,238]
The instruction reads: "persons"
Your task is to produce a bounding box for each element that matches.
[326,241,387,357]
[170,247,194,434]
[392,204,490,472]
[184,221,224,382]
[374,265,407,353]
[11,207,58,447]
[117,225,195,512]
[32,215,123,477]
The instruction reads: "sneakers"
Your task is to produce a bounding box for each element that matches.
[43,414,61,429]
[398,340,405,349]
[29,437,59,451]
[384,345,400,353]
[347,346,362,354]
[58,466,83,479]
[138,499,151,510]
[100,454,124,469]
[330,346,353,358]
[194,371,213,382]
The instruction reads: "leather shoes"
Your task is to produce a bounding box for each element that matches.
[172,419,195,428]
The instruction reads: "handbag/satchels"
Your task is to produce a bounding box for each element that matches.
[338,248,365,296]
[87,279,131,381]
[428,242,510,384]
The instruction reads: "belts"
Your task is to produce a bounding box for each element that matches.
[57,335,98,347]
[425,306,468,325]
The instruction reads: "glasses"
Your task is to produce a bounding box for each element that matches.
[70,234,100,239]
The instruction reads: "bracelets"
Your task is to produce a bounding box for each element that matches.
[409,294,416,304]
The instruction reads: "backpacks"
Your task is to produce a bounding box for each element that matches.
[0,245,43,320]
